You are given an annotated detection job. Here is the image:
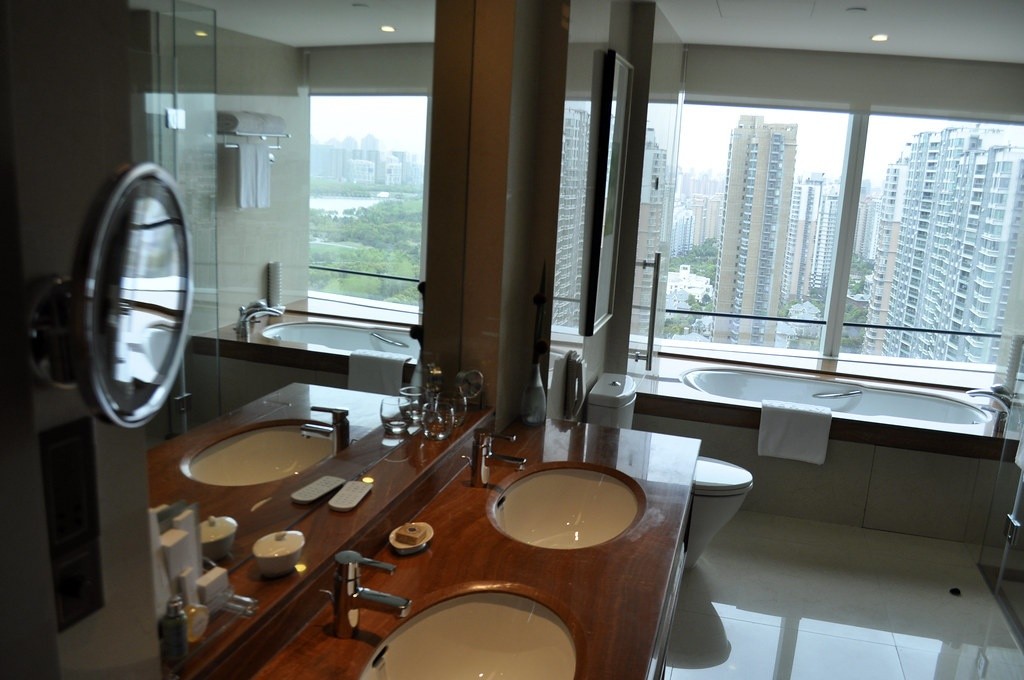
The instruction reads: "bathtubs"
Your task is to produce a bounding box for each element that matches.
[189,296,424,405]
[608,340,1015,546]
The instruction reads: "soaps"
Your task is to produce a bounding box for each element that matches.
[393,520,430,547]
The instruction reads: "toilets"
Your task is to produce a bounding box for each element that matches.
[586,370,754,576]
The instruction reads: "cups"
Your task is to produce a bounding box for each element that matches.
[421,402,456,441]
[379,397,414,433]
[433,394,467,427]
[398,386,430,423]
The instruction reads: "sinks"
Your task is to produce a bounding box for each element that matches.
[343,577,583,678]
[485,462,649,551]
[180,413,357,489]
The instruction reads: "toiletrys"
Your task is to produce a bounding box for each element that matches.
[148,519,247,665]
[147,500,209,584]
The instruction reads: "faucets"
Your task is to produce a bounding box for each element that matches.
[966,382,1014,412]
[322,548,416,642]
[468,426,529,488]
[300,402,351,459]
[235,302,284,332]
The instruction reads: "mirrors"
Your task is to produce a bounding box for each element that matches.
[1,2,484,679]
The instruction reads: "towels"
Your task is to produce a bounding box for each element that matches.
[757,397,833,467]
[346,347,412,396]
[253,142,273,210]
[235,142,257,214]
[217,109,264,137]
[247,111,286,136]
